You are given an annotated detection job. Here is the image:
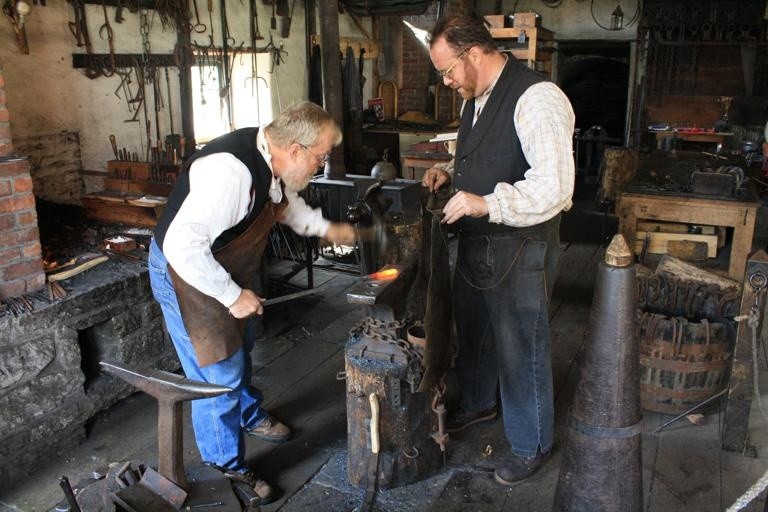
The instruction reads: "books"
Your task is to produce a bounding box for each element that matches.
[429,132,458,142]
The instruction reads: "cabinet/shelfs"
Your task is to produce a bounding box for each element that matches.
[80,159,184,226]
[482,27,555,83]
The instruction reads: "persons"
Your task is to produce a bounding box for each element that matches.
[148,102,357,505]
[421,13,577,488]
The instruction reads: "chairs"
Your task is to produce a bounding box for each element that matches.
[599,144,642,256]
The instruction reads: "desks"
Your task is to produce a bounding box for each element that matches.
[401,150,452,180]
[653,131,724,151]
[617,175,763,282]
[363,119,457,178]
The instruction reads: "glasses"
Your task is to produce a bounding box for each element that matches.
[438,47,471,77]
[303,144,330,165]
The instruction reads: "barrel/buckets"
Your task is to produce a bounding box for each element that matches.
[639,313,727,417]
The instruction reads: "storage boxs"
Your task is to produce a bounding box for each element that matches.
[513,12,541,30]
[482,15,513,30]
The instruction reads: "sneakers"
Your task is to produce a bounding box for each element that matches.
[240,472,274,505]
[446,403,496,433]
[495,446,552,486]
[245,411,292,444]
